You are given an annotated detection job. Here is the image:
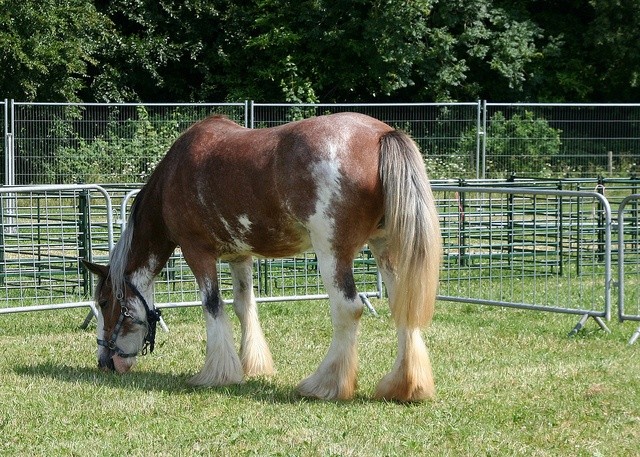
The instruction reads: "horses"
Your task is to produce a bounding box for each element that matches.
[77,111,445,404]
